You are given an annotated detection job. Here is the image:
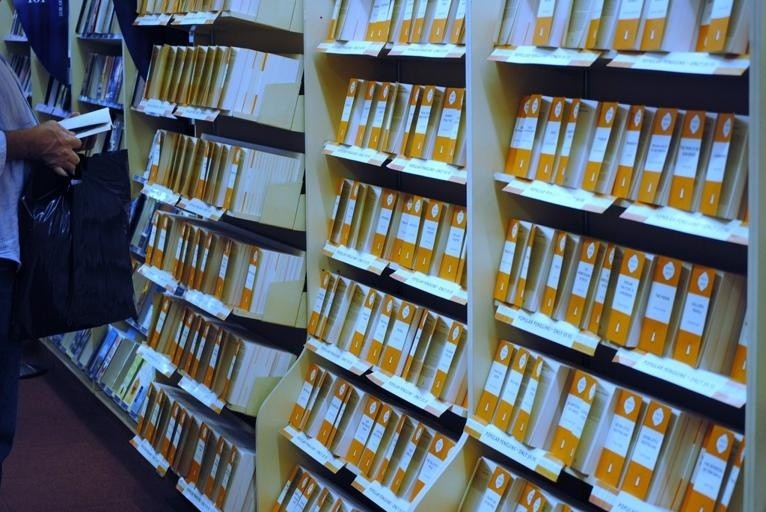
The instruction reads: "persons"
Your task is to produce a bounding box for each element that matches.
[0,51,83,463]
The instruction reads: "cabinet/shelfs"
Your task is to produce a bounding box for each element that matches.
[255,0,765,511]
[0,0,308,509]
